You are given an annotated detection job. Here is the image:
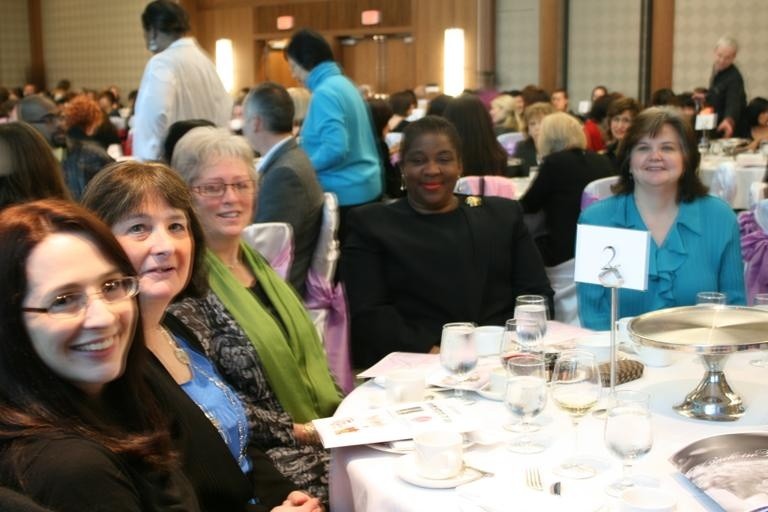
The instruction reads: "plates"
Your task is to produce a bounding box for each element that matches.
[616,489,676,510]
[392,465,467,490]
[360,440,471,458]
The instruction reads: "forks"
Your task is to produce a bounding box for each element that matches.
[523,466,542,493]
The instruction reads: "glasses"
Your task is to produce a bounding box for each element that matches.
[25,111,67,127]
[190,178,257,197]
[20,275,143,322]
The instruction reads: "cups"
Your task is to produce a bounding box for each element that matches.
[694,289,725,308]
[410,423,464,482]
[613,316,628,347]
[631,337,675,368]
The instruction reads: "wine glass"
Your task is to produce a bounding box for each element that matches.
[750,293,768,368]
[605,389,654,494]
[437,290,604,481]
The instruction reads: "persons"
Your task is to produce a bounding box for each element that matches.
[1,118,356,512]
[1,1,381,307]
[348,32,767,382]
